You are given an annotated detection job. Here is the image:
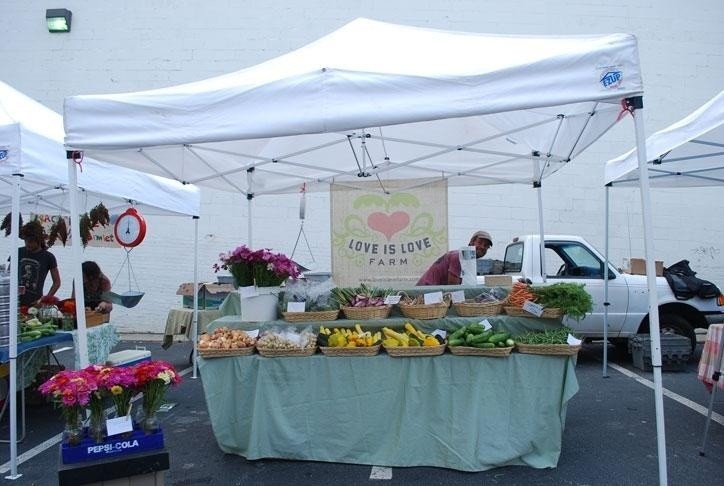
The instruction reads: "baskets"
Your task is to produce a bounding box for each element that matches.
[452,300,506,316]
[340,305,393,320]
[257,346,316,358]
[200,345,254,358]
[516,343,585,356]
[397,304,452,321]
[281,310,340,322]
[384,345,445,358]
[448,345,515,357]
[502,306,562,318]
[320,346,381,356]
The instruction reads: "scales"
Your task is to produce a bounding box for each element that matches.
[102,208,146,307]
[290,196,331,283]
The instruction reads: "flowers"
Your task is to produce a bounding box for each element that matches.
[134,359,182,416]
[211,242,255,272]
[36,368,98,429]
[96,366,140,415]
[60,301,75,315]
[252,247,302,279]
[38,295,58,306]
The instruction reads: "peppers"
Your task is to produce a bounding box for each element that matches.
[515,327,578,345]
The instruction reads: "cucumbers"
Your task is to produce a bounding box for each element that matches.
[448,323,514,348]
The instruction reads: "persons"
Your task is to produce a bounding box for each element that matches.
[416,231,493,286]
[71,261,112,314]
[8,222,60,307]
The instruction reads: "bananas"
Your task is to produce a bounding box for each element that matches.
[319,323,444,348]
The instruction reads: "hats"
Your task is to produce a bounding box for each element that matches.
[19,221,48,239]
[471,231,495,248]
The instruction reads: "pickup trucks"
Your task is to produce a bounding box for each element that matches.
[301,233,724,363]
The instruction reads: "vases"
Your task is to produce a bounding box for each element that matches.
[143,410,161,430]
[39,305,58,328]
[228,261,254,288]
[61,313,74,332]
[62,423,83,447]
[88,411,107,443]
[253,264,285,287]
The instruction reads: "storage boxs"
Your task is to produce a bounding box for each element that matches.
[621,257,664,277]
[491,260,504,274]
[106,348,151,367]
[627,333,692,372]
[476,258,496,275]
[175,282,236,310]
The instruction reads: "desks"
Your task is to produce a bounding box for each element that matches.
[694,321,723,457]
[54,441,169,486]
[0,322,111,444]
[161,308,224,363]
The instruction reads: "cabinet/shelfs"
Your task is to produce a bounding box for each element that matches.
[195,283,584,473]
